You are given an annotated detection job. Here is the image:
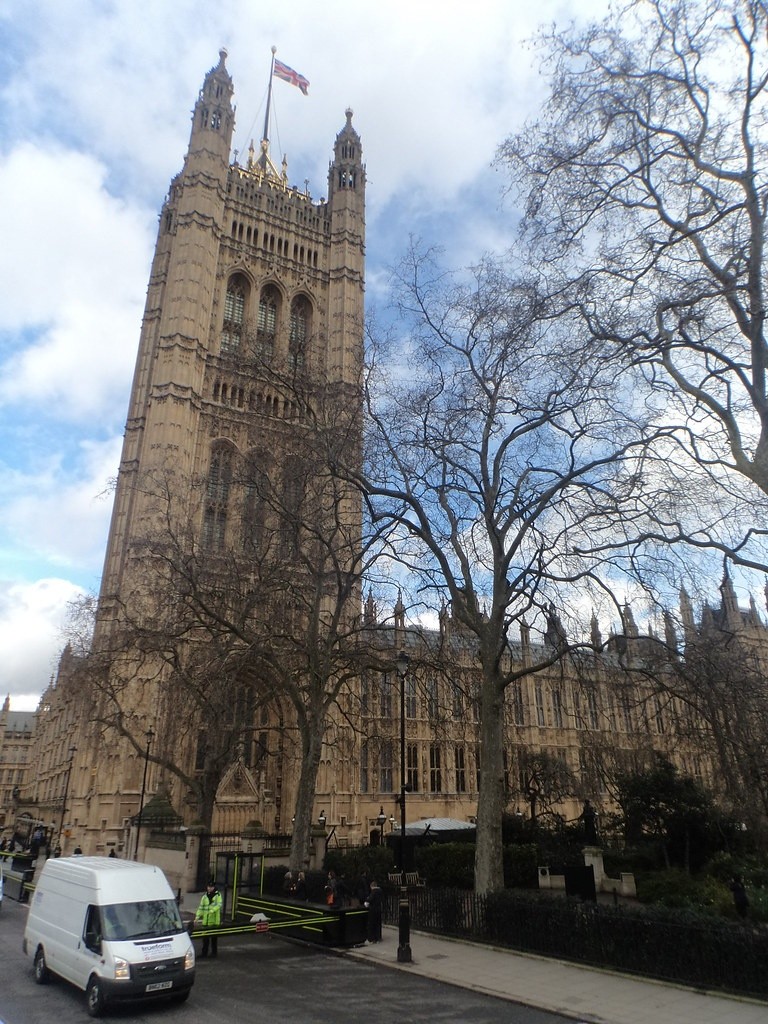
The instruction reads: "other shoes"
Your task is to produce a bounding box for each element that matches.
[364,939,374,945]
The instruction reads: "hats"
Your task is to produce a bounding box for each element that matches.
[207,881,216,887]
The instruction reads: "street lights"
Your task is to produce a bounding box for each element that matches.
[132,724,156,863]
[51,745,77,857]
[391,651,419,961]
[376,806,387,845]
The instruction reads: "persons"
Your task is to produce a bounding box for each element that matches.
[45,844,51,859]
[54,844,62,858]
[284,872,308,901]
[109,849,118,858]
[323,870,350,906]
[578,799,597,839]
[0,837,14,862]
[74,845,82,854]
[194,881,223,958]
[364,881,385,945]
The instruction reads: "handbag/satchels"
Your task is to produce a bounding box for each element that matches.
[326,894,333,904]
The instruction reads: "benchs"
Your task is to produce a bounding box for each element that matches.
[388,872,427,891]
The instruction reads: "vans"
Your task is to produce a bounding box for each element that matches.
[22,853,194,1015]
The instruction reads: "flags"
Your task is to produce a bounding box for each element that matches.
[273,58,310,96]
